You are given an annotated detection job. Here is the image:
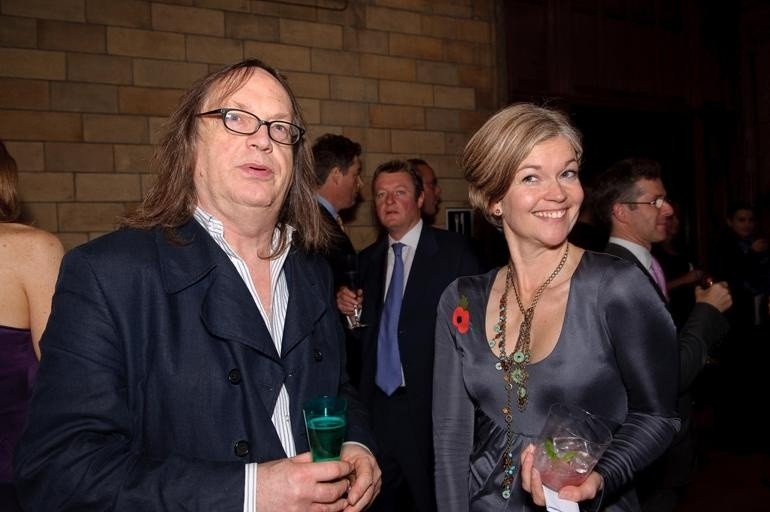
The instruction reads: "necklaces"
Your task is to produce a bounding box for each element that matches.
[491,238,572,500]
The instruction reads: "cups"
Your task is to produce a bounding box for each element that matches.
[532,401,614,492]
[301,392,351,462]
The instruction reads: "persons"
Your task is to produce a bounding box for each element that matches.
[714,202,769,413]
[332,159,478,511]
[567,158,605,253]
[14,56,385,512]
[0,145,66,512]
[407,160,444,226]
[276,133,363,367]
[657,204,696,321]
[588,160,732,510]
[430,102,683,510]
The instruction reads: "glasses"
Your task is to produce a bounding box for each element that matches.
[613,194,669,209]
[192,106,305,148]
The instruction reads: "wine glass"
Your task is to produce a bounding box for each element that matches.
[341,251,369,330]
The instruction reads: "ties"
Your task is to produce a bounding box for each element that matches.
[373,240,416,401]
